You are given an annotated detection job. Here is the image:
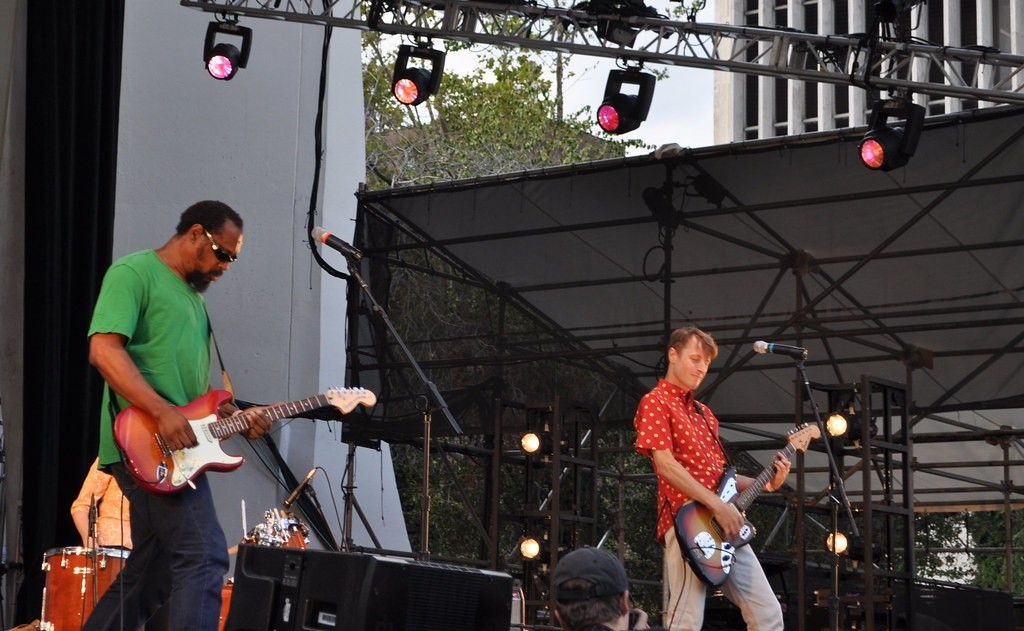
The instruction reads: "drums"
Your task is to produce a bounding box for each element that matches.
[41,545,146,631]
[246,519,307,550]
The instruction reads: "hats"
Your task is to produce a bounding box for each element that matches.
[553,546,629,601]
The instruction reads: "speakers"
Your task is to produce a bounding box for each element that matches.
[224,542,514,631]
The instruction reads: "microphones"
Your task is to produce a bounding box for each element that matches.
[89,493,96,537]
[753,340,807,358]
[283,469,318,509]
[311,227,364,260]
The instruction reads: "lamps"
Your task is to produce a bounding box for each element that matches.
[825,410,851,438]
[521,431,553,455]
[518,537,541,561]
[598,69,657,134]
[858,100,925,172]
[824,531,849,554]
[201,13,249,79]
[388,35,444,106]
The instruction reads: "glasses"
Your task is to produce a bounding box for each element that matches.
[203,228,238,263]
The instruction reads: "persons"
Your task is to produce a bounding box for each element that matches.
[70,454,133,551]
[81,200,283,631]
[552,547,651,631]
[633,327,792,631]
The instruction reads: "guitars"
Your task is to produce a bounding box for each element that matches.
[112,384,378,497]
[673,422,822,589]
